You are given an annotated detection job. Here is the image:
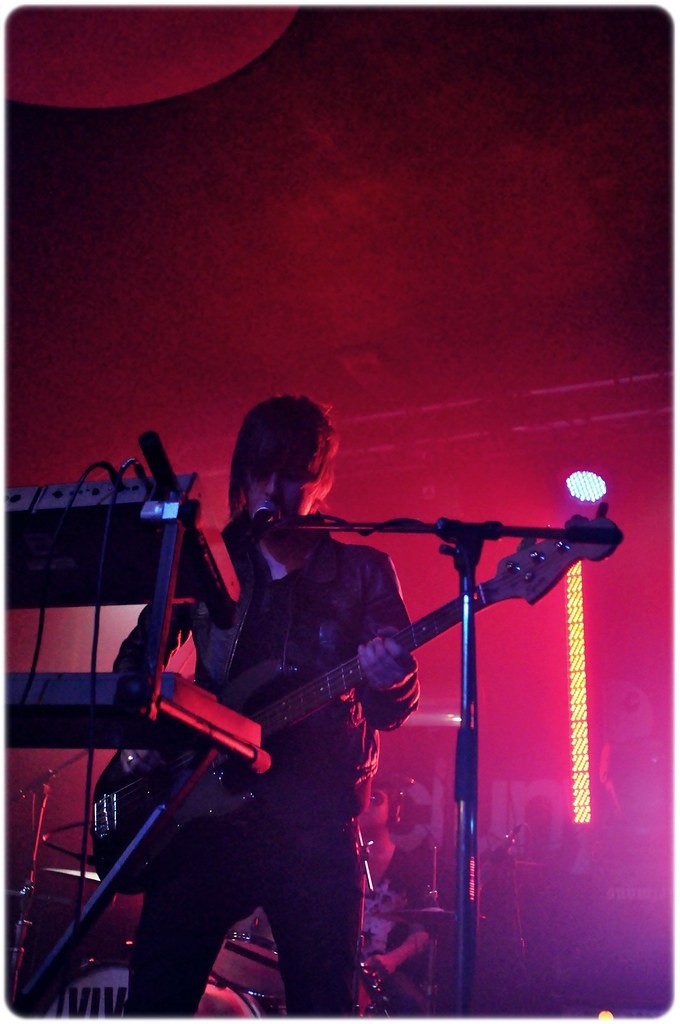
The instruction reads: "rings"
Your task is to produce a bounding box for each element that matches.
[126,755,137,764]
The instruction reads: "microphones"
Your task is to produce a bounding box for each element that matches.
[237,501,282,544]
[493,823,523,859]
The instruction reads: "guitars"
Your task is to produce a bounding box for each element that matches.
[86,500,627,895]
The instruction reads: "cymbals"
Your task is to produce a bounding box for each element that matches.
[377,896,488,930]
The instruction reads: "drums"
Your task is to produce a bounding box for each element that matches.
[210,928,287,1000]
[37,953,270,1019]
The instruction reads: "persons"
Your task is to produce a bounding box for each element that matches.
[111,391,422,1018]
[357,777,440,1018]
